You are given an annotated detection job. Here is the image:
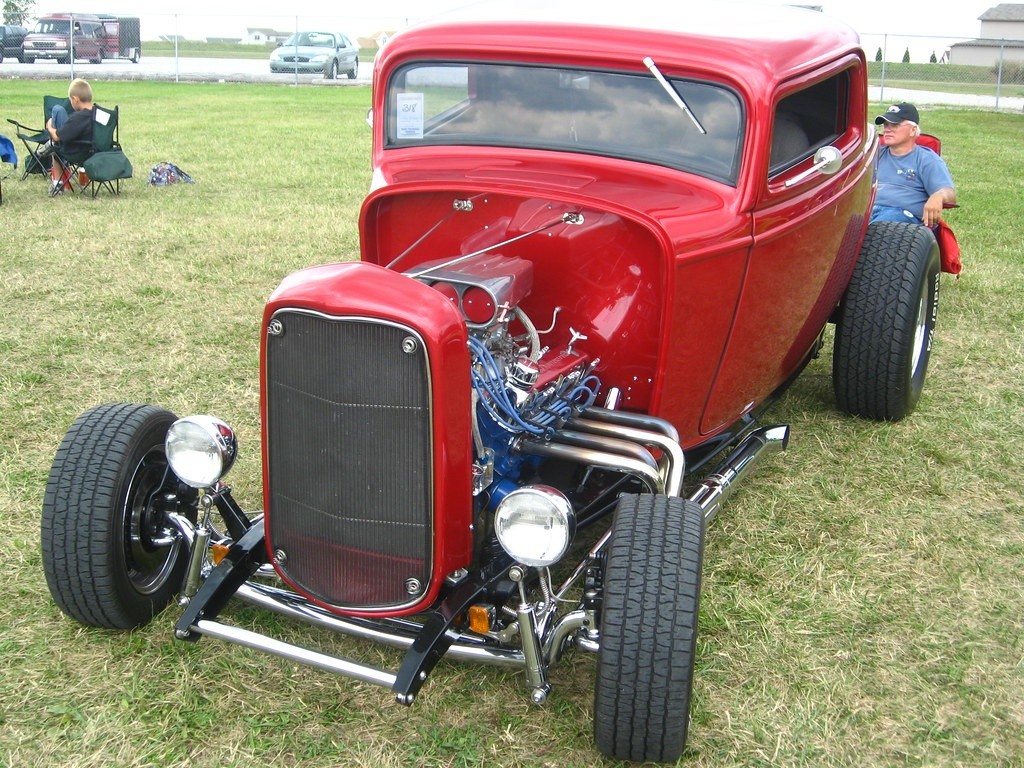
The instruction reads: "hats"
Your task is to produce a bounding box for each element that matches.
[874,101,919,125]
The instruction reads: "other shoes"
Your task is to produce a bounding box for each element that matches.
[36,140,55,158]
[46,181,66,196]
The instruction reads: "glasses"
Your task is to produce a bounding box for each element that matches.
[881,122,910,131]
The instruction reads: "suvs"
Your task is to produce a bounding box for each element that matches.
[21,15,107,63]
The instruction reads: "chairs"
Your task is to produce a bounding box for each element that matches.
[877,133,961,280]
[49,103,120,199]
[6,95,74,180]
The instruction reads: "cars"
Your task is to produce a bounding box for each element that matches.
[0,26,37,64]
[42,0,943,768]
[269,31,359,80]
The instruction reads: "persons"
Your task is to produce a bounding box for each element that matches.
[36,78,93,193]
[869,103,956,227]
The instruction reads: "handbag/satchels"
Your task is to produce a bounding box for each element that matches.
[147,161,196,187]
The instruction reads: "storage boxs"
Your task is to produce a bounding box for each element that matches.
[52,166,88,190]
